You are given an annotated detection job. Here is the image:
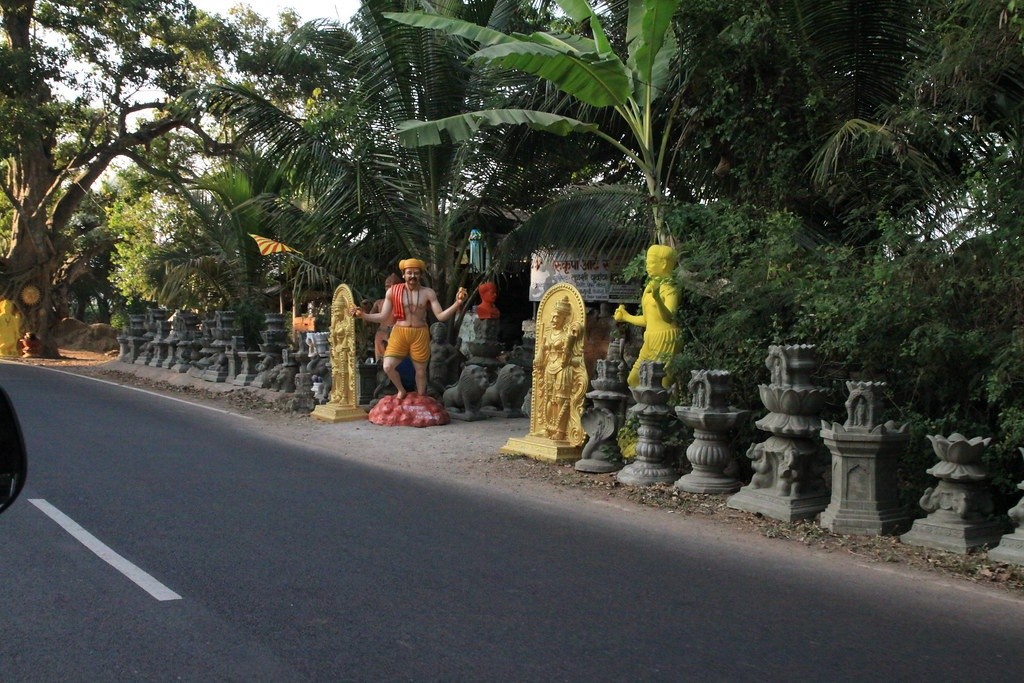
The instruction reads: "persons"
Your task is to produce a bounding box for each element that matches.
[614,245,685,388]
[350,258,468,405]
[328,273,402,406]
[477,283,500,319]
[533,295,582,441]
[429,322,460,393]
